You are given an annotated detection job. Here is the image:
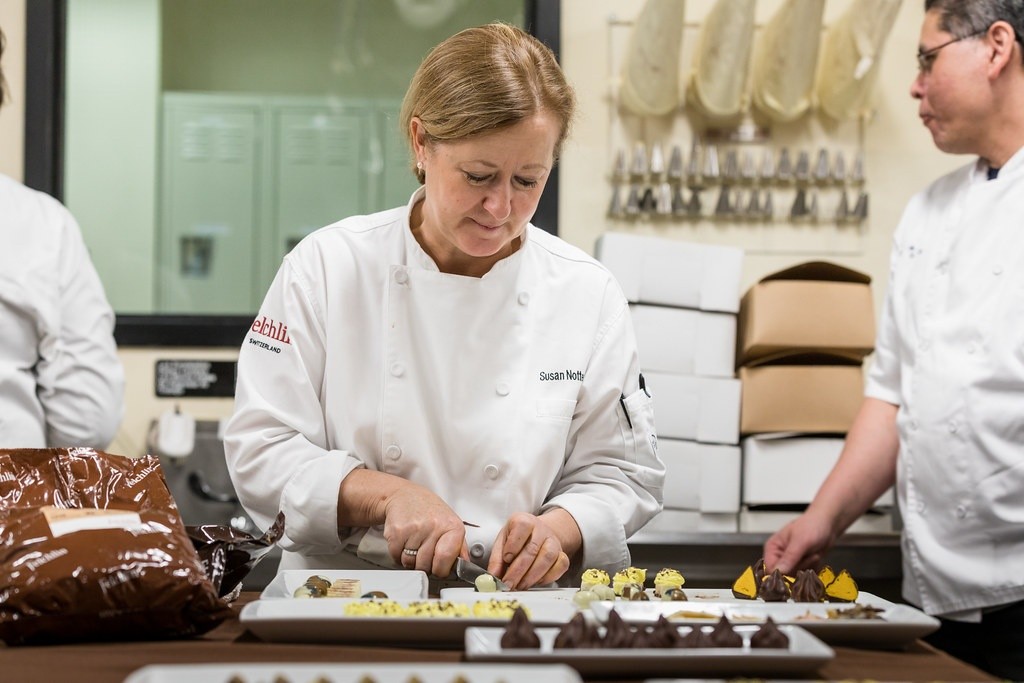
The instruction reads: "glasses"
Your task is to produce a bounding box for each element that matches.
[918,28,988,74]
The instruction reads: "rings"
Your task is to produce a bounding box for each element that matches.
[401,550,419,556]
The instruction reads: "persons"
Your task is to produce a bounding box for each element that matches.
[763,0,1024,683]
[221,22,666,594]
[0,27,126,449]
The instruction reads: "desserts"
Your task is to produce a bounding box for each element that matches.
[296,570,881,651]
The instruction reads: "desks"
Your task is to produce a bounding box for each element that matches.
[0,592,1004,683]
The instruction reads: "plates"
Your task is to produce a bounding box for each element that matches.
[239,598,578,638]
[258,569,429,599]
[440,587,581,597]
[591,589,939,641]
[465,625,833,674]
[125,663,581,683]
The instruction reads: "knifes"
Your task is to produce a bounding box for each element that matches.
[453,556,505,590]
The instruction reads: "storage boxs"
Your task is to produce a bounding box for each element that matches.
[737,349,866,435]
[593,232,742,314]
[737,260,876,359]
[743,436,895,512]
[627,304,737,378]
[644,374,740,445]
[656,439,741,514]
